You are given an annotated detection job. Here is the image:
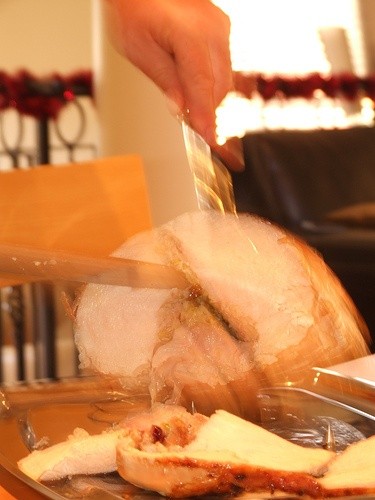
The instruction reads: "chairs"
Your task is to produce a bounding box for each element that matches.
[0,154,157,379]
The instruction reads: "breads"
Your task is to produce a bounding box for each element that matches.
[17,208,374,499]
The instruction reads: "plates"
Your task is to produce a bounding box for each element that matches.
[0,368,374,500]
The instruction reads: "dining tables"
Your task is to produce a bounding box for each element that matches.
[0,377,374,500]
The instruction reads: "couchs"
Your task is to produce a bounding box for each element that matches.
[232,125,375,353]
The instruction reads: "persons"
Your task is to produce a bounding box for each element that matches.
[101,0,234,148]
